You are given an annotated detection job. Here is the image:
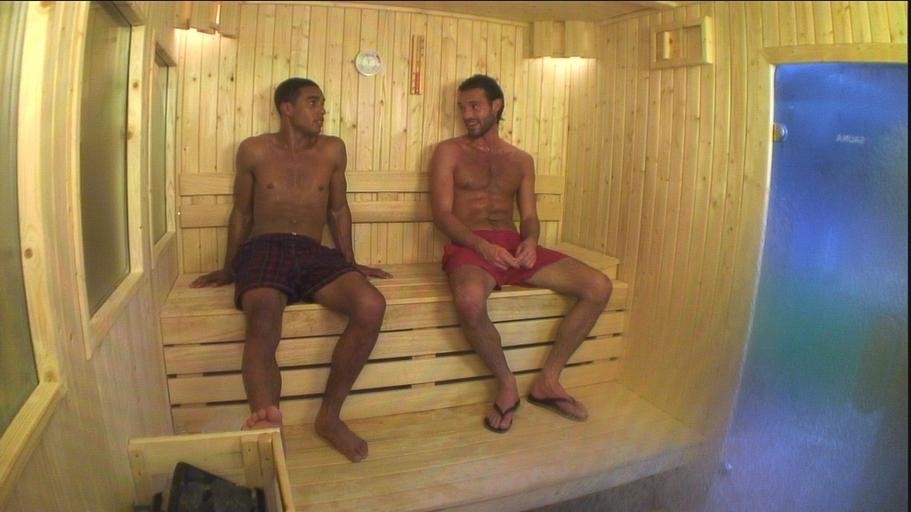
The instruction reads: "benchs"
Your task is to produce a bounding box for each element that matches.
[159,173,628,434]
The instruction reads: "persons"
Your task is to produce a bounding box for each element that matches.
[189,77,393,464]
[427,73,611,433]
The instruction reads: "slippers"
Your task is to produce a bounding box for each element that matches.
[483,394,521,435]
[525,388,590,423]
